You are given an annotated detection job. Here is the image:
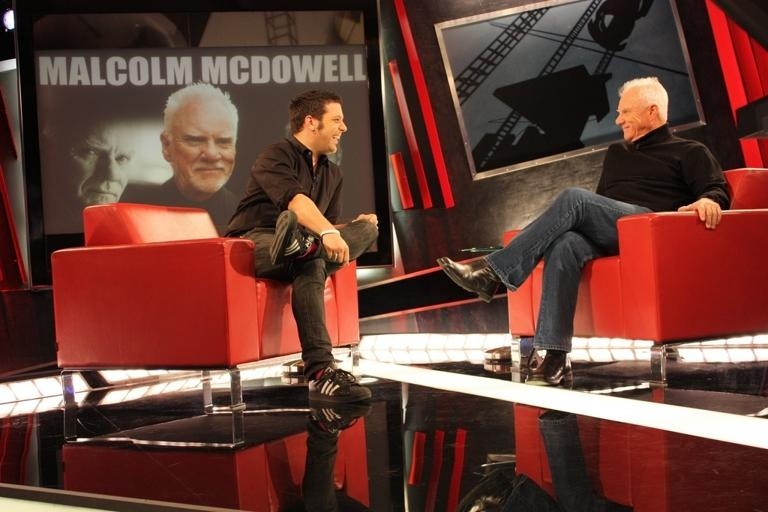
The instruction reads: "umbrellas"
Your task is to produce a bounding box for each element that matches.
[525,352,573,386]
[435,257,500,304]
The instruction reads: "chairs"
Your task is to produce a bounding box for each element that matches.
[307,361,372,404]
[268,210,315,266]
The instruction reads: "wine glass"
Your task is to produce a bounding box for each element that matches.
[332,253,338,261]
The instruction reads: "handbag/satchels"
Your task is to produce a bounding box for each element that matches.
[320,230,340,238]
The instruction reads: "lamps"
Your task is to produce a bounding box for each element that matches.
[513,386,768,512]
[51,202,358,413]
[502,167,768,386]
[60,412,371,512]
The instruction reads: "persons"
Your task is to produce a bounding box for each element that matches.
[277,403,376,512]
[221,88,380,404]
[435,75,732,386]
[143,81,241,238]
[454,409,638,512]
[39,109,133,233]
